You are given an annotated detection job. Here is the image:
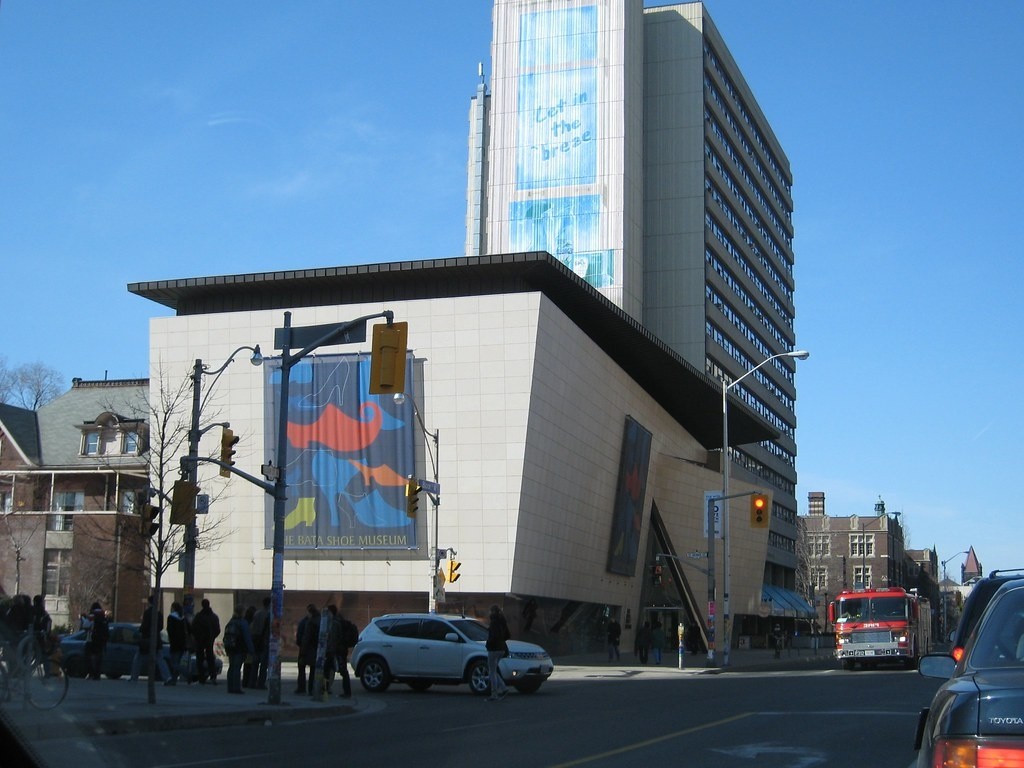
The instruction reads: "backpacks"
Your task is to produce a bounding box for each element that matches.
[336,619,358,648]
[223,621,241,654]
[91,619,109,644]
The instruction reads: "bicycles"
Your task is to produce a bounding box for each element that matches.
[0,629,70,712]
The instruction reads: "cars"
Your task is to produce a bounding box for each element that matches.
[59,622,223,681]
[908,579,1024,768]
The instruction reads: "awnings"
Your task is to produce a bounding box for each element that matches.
[762,585,819,620]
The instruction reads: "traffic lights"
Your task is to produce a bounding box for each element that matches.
[406,480,422,519]
[170,481,201,525]
[139,504,160,537]
[449,561,461,583]
[220,428,239,478]
[751,495,769,528]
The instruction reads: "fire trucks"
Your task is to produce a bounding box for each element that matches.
[830,587,932,671]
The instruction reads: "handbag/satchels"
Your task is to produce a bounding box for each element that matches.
[184,633,195,653]
[502,643,509,658]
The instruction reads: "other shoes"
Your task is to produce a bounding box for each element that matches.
[500,689,511,698]
[484,697,498,702]
[227,689,244,694]
[163,678,175,686]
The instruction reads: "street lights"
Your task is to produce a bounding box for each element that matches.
[393,392,438,613]
[863,512,901,589]
[722,350,810,665]
[943,551,969,642]
[179,345,263,681]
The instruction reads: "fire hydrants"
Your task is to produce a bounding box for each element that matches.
[48,648,62,678]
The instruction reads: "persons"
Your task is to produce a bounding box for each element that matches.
[687,620,700,655]
[79,602,109,681]
[162,602,192,686]
[635,621,668,665]
[294,604,359,699]
[607,614,621,663]
[223,595,270,693]
[5,594,52,681]
[192,599,221,685]
[483,604,511,703]
[128,595,172,685]
[773,624,783,655]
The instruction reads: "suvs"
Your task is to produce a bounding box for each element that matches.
[350,613,554,697]
[949,569,1024,668]
